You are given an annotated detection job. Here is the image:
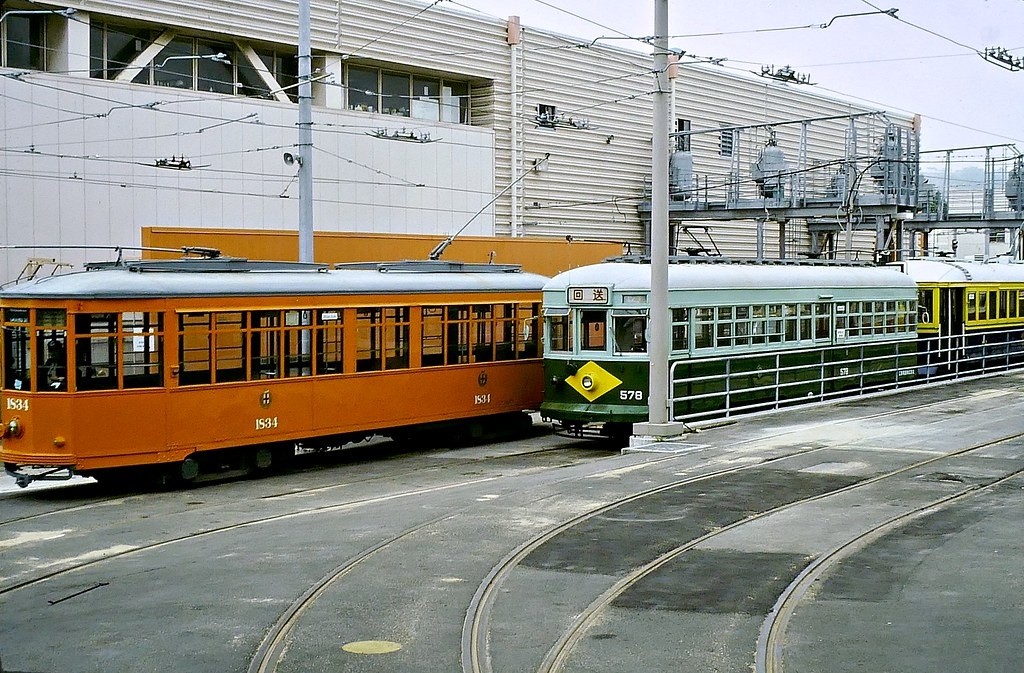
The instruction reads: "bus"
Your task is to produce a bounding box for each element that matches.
[541,253,918,446]
[889,255,1024,371]
[0,247,553,490]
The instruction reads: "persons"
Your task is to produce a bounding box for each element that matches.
[45,340,82,382]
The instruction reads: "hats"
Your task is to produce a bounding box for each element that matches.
[47,340,62,351]
